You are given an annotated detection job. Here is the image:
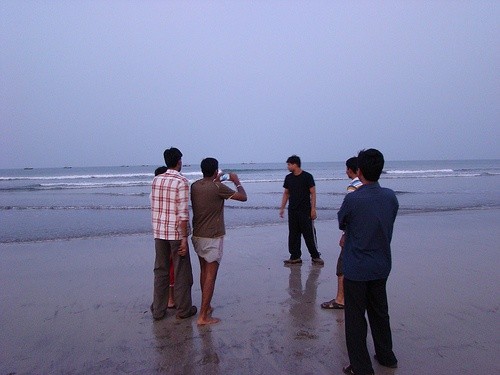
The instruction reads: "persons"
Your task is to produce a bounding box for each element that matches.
[155,166,176,309]
[279,155,324,264]
[190,158,248,325]
[321,156,364,310]
[150,148,198,320]
[337,148,399,375]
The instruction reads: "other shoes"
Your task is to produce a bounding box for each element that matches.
[283,257,325,264]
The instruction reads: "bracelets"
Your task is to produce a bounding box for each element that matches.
[182,235,187,237]
[236,185,242,188]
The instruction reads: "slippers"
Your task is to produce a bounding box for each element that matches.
[344,355,398,375]
[151,301,198,320]
[321,299,345,309]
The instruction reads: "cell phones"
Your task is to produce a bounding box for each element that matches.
[220,174,230,181]
